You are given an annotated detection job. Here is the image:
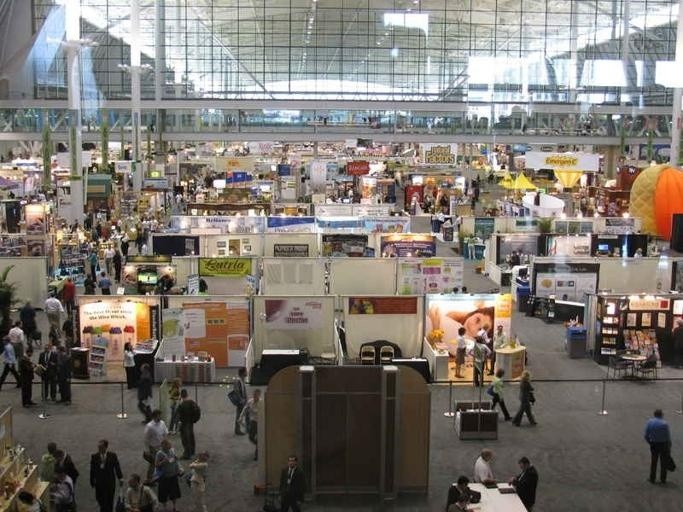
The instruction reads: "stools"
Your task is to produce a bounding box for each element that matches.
[319,351,336,365]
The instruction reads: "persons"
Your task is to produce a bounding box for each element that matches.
[674,320,683,369]
[136,364,153,424]
[446,448,538,511]
[40,378,209,511]
[71,205,165,294]
[468,234,476,260]
[511,252,520,268]
[645,409,671,483]
[455,325,538,427]
[0,278,83,408]
[172,172,221,207]
[234,367,264,460]
[534,192,540,207]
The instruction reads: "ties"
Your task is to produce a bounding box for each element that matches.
[46,351,49,361]
[288,469,292,478]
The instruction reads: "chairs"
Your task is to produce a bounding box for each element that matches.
[379,346,393,366]
[360,345,375,365]
[602,348,657,380]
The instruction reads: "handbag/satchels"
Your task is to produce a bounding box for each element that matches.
[115,496,125,512]
[31,329,41,340]
[668,457,676,472]
[487,386,494,396]
[191,402,201,423]
[227,391,241,406]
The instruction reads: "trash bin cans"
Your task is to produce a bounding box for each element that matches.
[442,226,453,241]
[567,327,587,359]
[516,287,530,312]
[432,220,440,233]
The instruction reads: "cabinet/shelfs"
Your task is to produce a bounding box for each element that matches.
[0,447,49,512]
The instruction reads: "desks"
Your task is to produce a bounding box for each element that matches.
[258,349,308,385]
[495,345,525,380]
[451,480,528,512]
[431,347,449,380]
[389,357,429,381]
[69,346,90,379]
[133,338,158,381]
[156,356,214,383]
[531,296,585,323]
